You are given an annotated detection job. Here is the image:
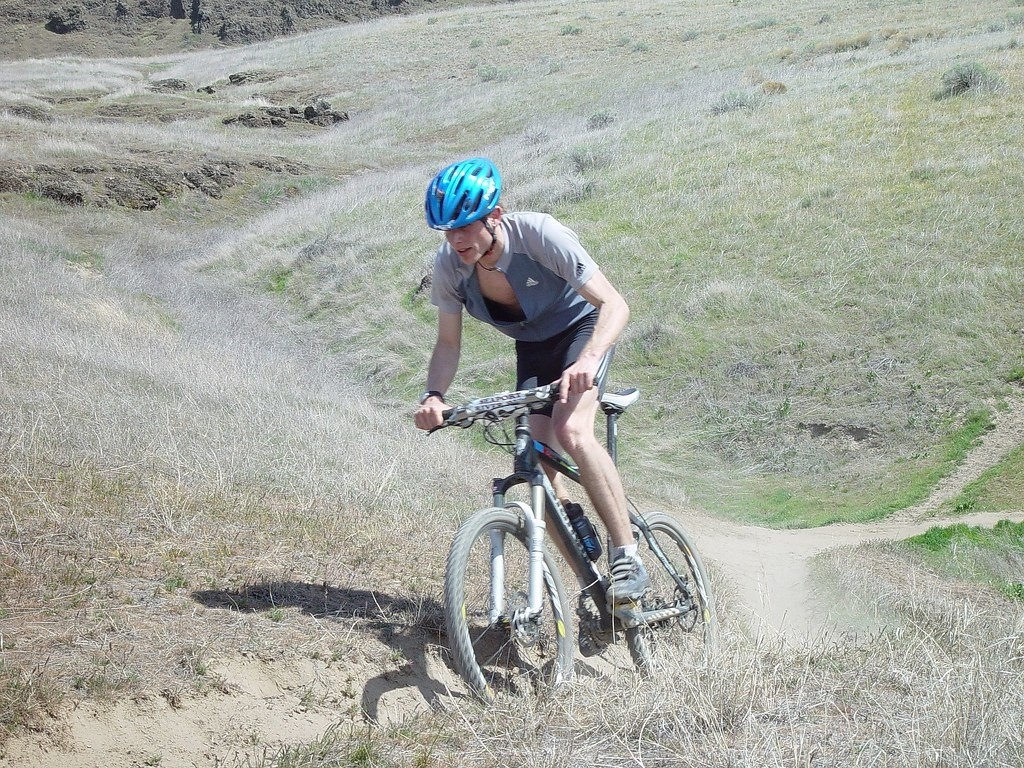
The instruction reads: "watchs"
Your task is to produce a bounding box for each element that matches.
[420,391,445,407]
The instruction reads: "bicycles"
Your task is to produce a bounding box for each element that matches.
[425,341,717,709]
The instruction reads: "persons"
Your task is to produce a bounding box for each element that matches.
[415,159,648,657]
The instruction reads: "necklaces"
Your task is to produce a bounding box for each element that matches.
[478,261,496,271]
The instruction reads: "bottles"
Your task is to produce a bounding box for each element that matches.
[562,499,602,561]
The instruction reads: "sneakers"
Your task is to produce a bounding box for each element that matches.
[578,573,612,657]
[604,549,653,603]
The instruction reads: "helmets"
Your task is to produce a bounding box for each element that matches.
[425,157,502,230]
